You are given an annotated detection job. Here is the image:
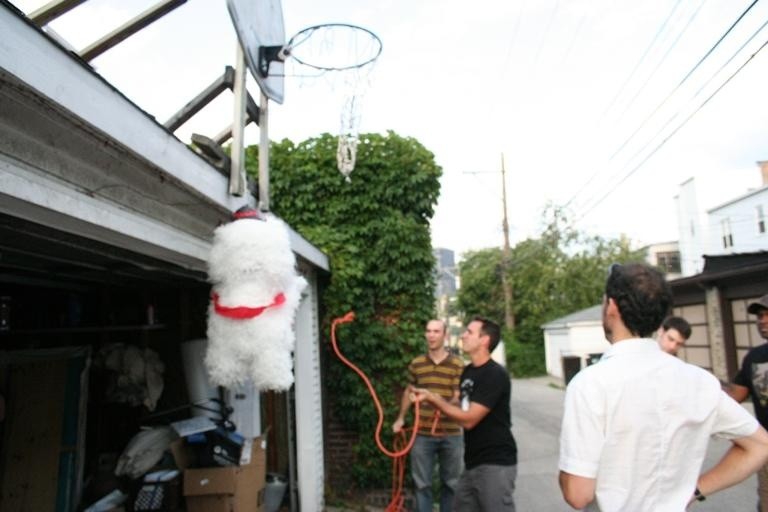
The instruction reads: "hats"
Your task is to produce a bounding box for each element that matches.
[747,294,767,313]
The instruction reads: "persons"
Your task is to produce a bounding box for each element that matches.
[650,314,693,358]
[705,293,768,512]
[389,317,468,512]
[407,316,521,512]
[552,260,768,512]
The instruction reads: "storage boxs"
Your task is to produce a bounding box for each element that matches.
[170,437,267,512]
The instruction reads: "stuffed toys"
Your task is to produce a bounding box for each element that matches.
[199,203,309,398]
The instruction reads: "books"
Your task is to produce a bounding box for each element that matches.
[170,415,219,438]
[132,467,181,511]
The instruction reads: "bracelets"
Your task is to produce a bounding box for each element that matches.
[694,488,706,501]
